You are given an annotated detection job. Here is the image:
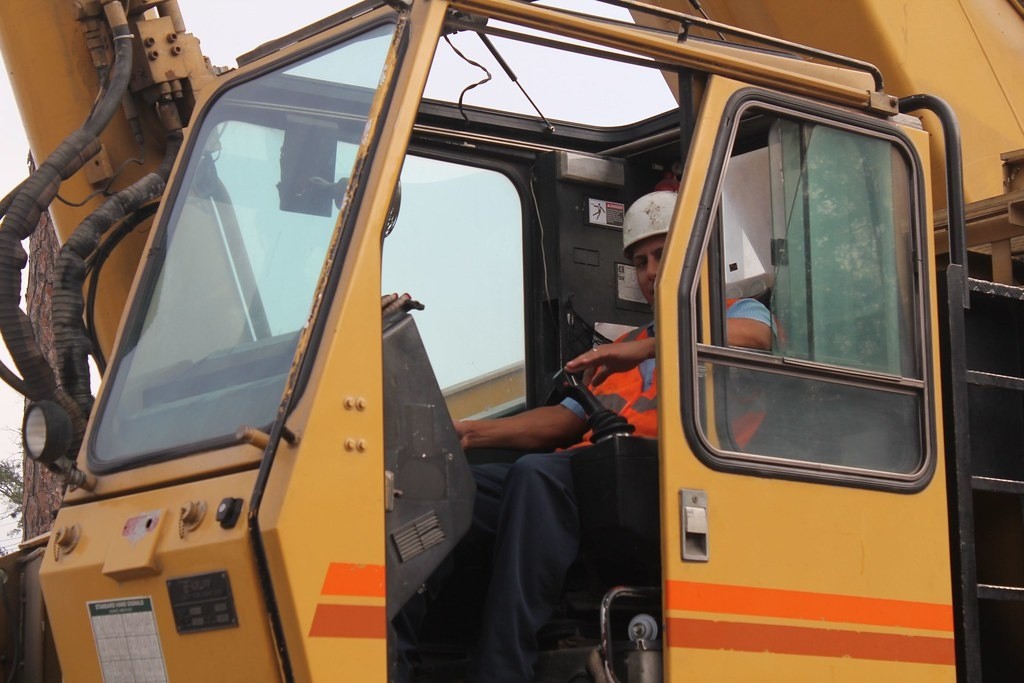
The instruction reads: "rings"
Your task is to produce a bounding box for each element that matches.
[592,348,597,352]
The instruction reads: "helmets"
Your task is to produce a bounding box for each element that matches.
[621,190,679,260]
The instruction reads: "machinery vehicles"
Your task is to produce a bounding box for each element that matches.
[0,0,1024,683]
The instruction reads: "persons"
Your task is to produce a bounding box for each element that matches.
[392,189,787,683]
